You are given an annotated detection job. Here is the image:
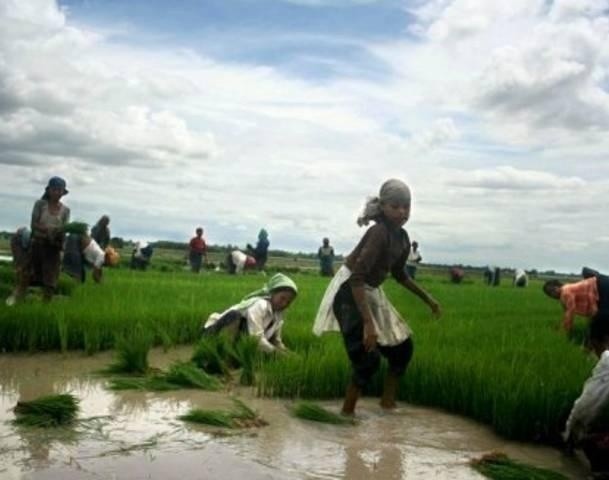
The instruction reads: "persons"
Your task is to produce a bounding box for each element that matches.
[513,268,528,287]
[311,178,443,417]
[131,240,153,270]
[448,266,465,282]
[317,238,335,278]
[185,227,209,273]
[407,240,421,278]
[223,229,269,276]
[203,273,300,375]
[484,264,499,286]
[6,178,120,306]
[542,267,609,479]
